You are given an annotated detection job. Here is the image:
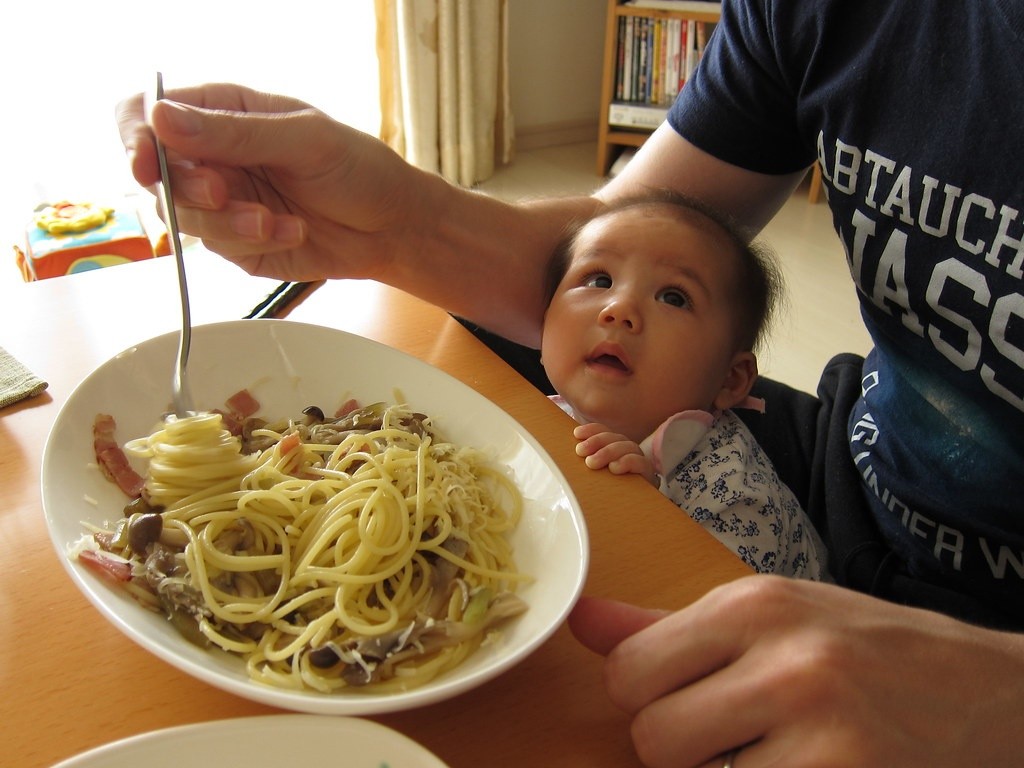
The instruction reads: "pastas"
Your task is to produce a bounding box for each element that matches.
[111,407,537,696]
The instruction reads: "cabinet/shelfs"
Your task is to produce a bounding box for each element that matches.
[598,1,824,206]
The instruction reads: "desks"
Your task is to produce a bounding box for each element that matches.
[0,246,764,768]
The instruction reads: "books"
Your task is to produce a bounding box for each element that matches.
[615,15,706,106]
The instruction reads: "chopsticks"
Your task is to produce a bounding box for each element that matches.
[241,281,310,319]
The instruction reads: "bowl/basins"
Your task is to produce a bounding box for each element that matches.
[39,319,591,717]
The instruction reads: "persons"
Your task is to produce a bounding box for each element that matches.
[540,191,831,581]
[114,0,1024,768]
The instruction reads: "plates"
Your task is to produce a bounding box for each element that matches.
[51,713,449,768]
